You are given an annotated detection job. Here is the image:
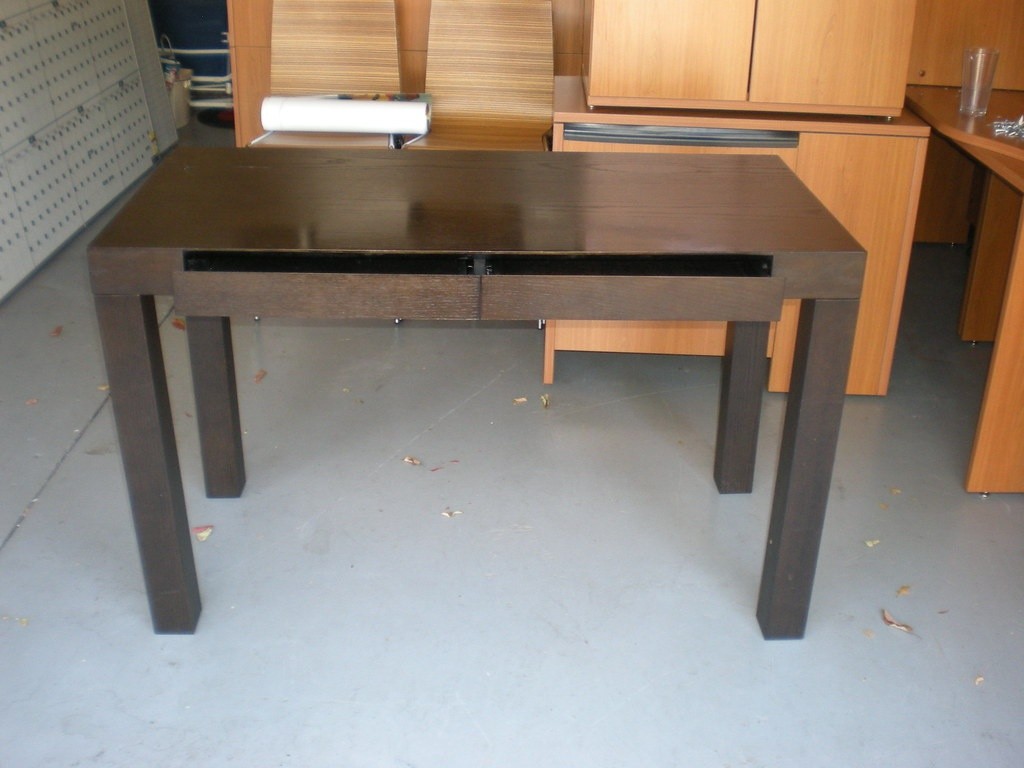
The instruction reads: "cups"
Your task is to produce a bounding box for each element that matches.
[961,47,1000,117]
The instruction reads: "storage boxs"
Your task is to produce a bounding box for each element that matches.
[168,68,191,130]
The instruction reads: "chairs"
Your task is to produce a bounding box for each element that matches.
[249,0,398,149]
[398,0,555,153]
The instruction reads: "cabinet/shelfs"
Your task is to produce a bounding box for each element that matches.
[544,77,933,396]
[585,0,917,123]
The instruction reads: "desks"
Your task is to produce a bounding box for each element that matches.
[905,84,1024,493]
[85,152,868,639]
